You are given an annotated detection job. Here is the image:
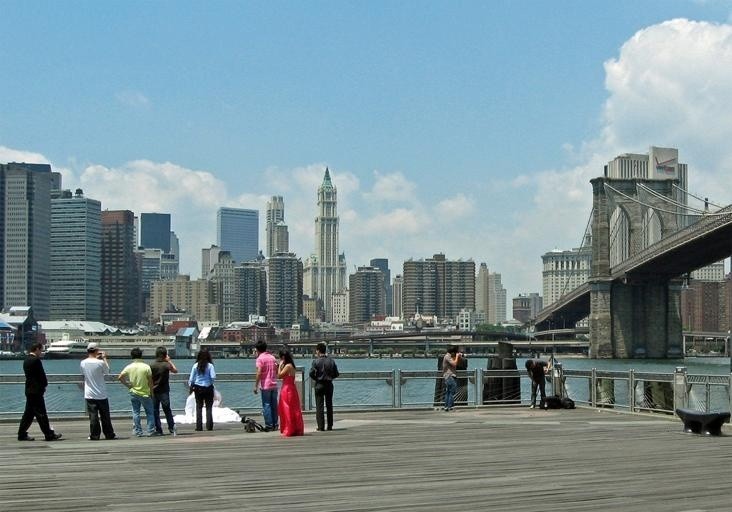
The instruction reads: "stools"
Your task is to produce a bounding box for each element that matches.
[674,406,732,436]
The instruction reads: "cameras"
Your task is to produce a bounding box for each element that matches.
[457,351,469,358]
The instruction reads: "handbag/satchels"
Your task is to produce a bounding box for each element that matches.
[241,416,262,432]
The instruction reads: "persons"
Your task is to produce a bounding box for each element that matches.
[276,346,304,437]
[252,339,279,431]
[80,343,120,441]
[188,350,217,431]
[309,343,340,431]
[525,360,551,409]
[148,346,178,436]
[441,345,463,411]
[118,347,159,438]
[17,342,63,441]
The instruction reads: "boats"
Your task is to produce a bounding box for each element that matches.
[45,340,88,355]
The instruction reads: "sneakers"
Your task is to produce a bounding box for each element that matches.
[88,436,99,440]
[18,434,34,441]
[46,434,62,440]
[106,435,119,439]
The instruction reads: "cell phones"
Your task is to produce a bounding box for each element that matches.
[98,351,102,356]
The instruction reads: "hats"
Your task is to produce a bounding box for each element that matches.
[87,343,96,350]
